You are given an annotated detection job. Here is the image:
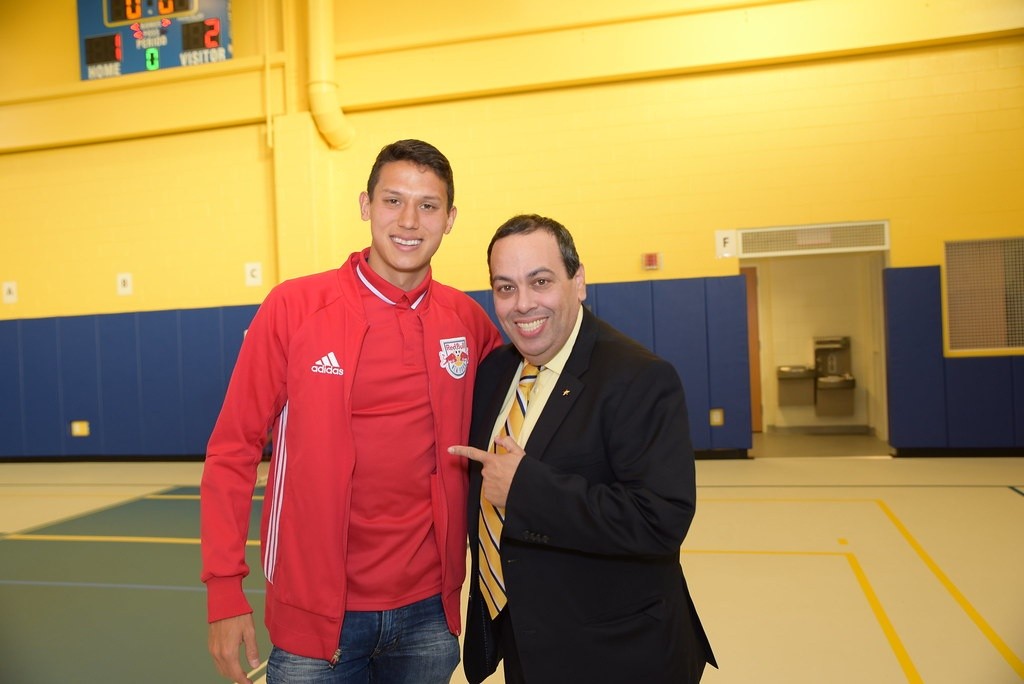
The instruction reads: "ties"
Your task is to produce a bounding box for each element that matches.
[478,355,547,621]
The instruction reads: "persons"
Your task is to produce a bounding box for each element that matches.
[200,138,507,684]
[447,213,717,684]
